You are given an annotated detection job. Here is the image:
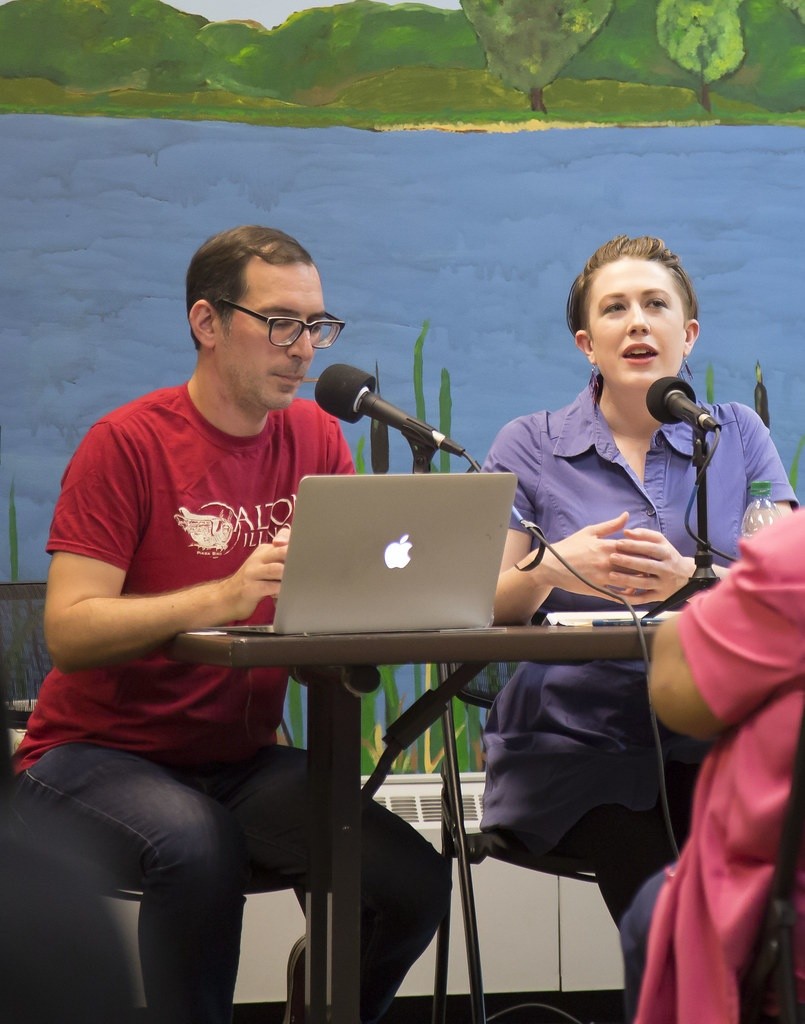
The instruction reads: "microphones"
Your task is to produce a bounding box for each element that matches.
[645,376,723,436]
[315,364,467,458]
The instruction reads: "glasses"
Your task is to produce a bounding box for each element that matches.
[214,298,346,348]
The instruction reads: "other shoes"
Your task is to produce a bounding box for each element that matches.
[284,933,307,1024]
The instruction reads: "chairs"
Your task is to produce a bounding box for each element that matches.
[741,717,805,1023]
[0,580,303,1024]
[431,657,601,1024]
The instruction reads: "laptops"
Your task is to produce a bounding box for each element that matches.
[184,471,521,634]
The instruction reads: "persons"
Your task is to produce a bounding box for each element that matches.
[476,236,798,926]
[16,227,451,1024]
[618,504,805,1024]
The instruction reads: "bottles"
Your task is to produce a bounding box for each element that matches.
[742,479,779,542]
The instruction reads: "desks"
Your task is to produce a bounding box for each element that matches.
[165,627,654,1023]
